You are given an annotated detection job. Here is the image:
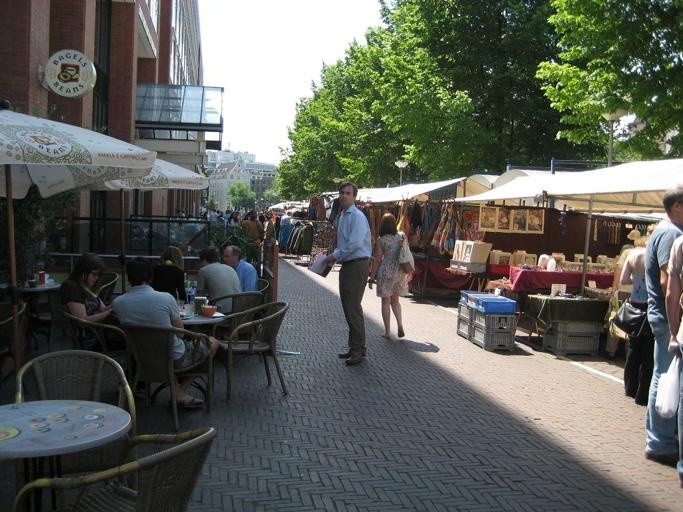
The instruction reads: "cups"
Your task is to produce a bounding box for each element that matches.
[38,271,45,285]
[194,297,207,313]
[175,301,184,311]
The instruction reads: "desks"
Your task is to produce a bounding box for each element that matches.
[2,277,61,352]
[408,254,616,358]
[150,305,228,405]
[2,400,131,509]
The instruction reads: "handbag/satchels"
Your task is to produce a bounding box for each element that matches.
[310,252,331,277]
[611,302,647,337]
[399,240,415,274]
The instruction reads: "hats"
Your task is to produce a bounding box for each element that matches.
[627,230,641,240]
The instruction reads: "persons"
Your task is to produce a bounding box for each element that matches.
[197,246,243,372]
[320,182,373,366]
[219,244,260,367]
[368,214,412,342]
[152,244,187,304]
[113,257,205,410]
[175,202,292,252]
[58,250,147,405]
[605,186,683,488]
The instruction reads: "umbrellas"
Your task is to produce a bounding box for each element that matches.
[0,108,157,394]
[89,157,209,293]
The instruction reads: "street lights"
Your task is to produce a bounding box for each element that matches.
[598,105,632,168]
[394,160,410,186]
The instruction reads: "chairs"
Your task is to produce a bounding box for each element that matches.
[95,271,119,312]
[123,321,216,425]
[17,347,137,508]
[209,291,262,368]
[207,302,287,403]
[11,427,218,510]
[32,290,62,352]
[1,300,27,388]
[58,310,147,407]
[258,279,269,340]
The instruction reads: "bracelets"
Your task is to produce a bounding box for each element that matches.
[368,280,375,284]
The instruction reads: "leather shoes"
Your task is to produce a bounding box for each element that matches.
[339,349,366,364]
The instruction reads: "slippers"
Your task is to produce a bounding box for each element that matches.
[171,397,204,408]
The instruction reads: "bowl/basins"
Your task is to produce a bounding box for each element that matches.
[200,305,217,316]
[27,279,37,287]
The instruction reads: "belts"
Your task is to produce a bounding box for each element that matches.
[343,257,368,263]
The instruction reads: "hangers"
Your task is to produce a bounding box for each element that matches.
[297,222,319,232]
